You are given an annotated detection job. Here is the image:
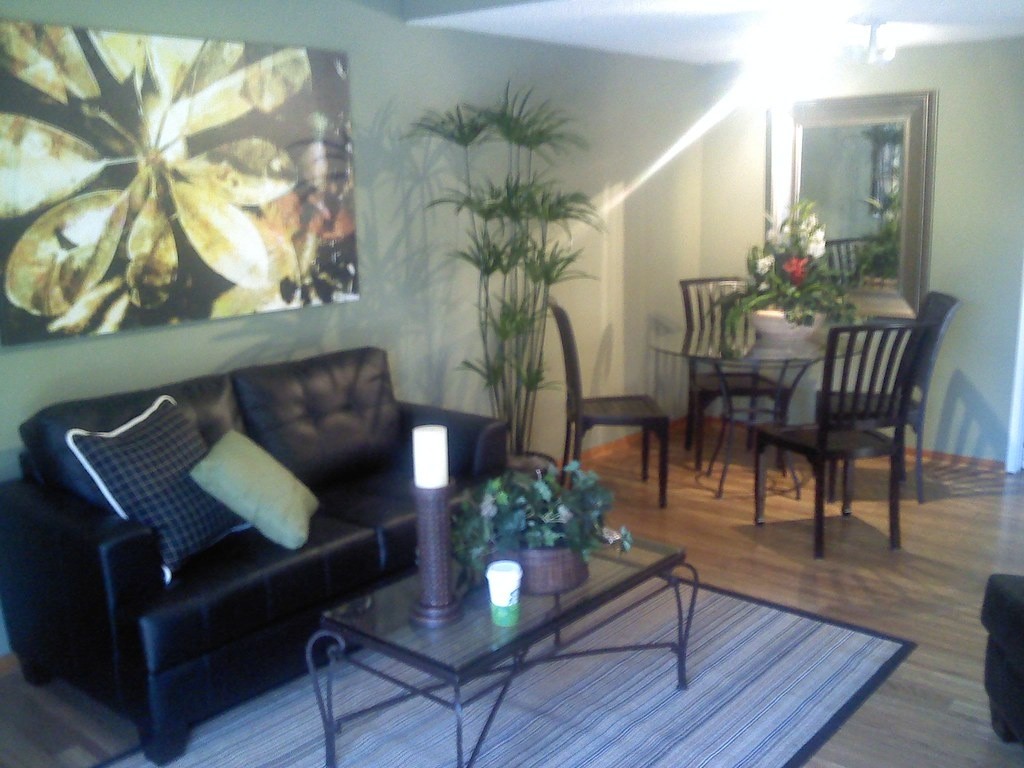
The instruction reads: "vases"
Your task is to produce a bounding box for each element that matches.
[752,310,827,345]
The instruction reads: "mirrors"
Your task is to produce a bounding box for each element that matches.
[764,87,939,324]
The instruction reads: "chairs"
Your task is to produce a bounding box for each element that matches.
[550,304,670,508]
[680,278,790,471]
[752,321,935,558]
[813,292,960,504]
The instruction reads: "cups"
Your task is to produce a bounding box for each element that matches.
[485,560,521,625]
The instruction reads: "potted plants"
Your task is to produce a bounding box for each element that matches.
[401,74,602,473]
[449,460,634,597]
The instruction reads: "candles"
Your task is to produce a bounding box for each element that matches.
[411,425,448,488]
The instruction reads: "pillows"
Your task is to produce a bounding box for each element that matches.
[190,429,321,552]
[66,394,254,585]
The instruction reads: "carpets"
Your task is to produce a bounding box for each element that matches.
[91,554,918,768]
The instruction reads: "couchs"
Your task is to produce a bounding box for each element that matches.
[0,344,512,766]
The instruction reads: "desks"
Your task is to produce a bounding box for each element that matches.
[650,326,874,504]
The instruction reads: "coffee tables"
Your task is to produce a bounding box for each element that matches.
[304,531,701,768]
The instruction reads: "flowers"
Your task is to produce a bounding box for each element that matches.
[709,194,857,327]
